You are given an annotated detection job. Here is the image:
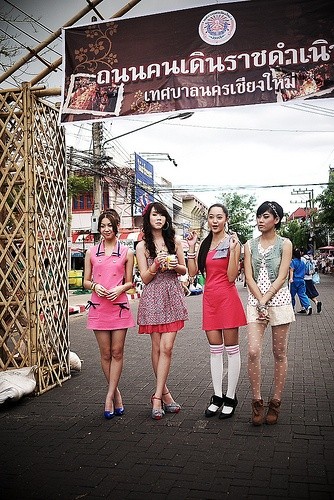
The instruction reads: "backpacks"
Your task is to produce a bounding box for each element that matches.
[303,256,314,275]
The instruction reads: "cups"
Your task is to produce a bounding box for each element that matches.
[167,255,176,269]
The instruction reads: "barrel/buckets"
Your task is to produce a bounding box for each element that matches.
[69,270,83,287]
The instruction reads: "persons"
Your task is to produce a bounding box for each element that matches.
[244,200,296,426]
[187,204,249,419]
[134,248,334,316]
[82,208,136,418]
[137,202,188,420]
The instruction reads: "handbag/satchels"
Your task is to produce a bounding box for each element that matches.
[312,272,320,284]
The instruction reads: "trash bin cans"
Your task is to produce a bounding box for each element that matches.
[68,270,83,289]
[198,273,204,286]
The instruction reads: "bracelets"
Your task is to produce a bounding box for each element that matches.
[187,253,196,259]
[267,285,277,295]
[90,282,97,291]
[149,270,156,276]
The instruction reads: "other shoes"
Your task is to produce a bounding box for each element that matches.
[297,310,306,313]
[104,400,115,418]
[114,401,125,415]
[219,394,238,419]
[252,398,265,426]
[265,398,282,424]
[307,306,312,316]
[205,394,224,417]
[317,301,321,312]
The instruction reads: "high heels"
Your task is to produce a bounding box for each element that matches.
[151,393,165,420]
[161,392,181,413]
